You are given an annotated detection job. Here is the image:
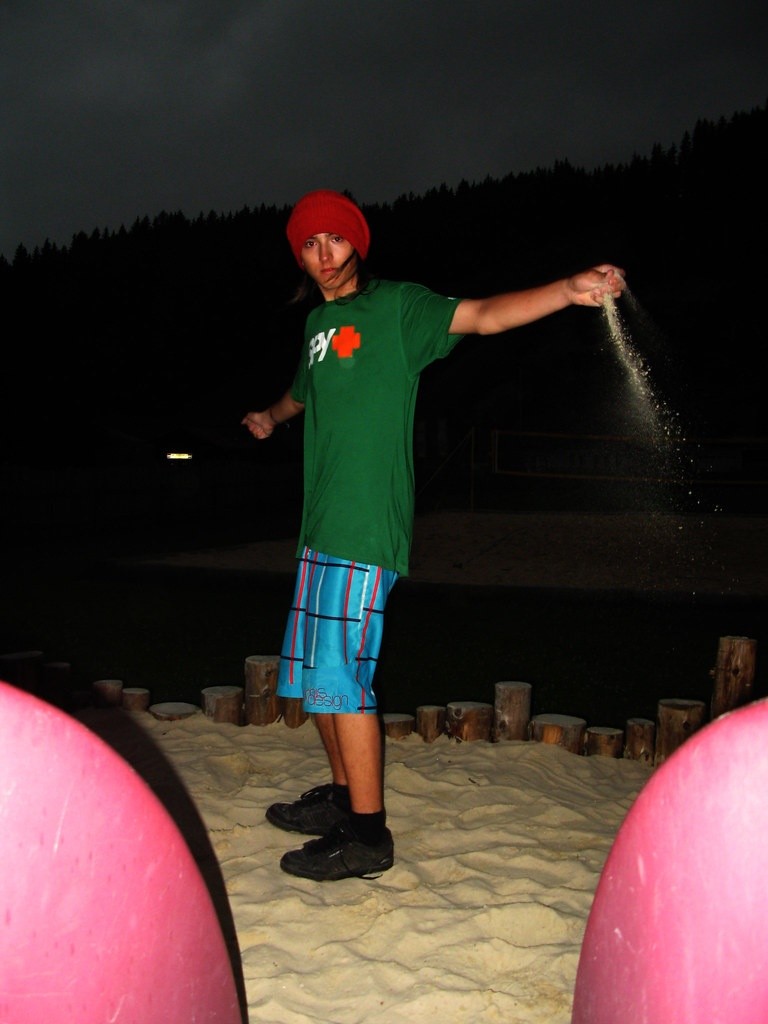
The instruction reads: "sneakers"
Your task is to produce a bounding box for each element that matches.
[280,824,395,882]
[265,787,347,835]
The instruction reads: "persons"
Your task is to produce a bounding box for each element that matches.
[240,188,629,882]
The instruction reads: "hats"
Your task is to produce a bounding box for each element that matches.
[285,191,370,266]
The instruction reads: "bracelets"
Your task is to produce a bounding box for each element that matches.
[270,406,281,427]
